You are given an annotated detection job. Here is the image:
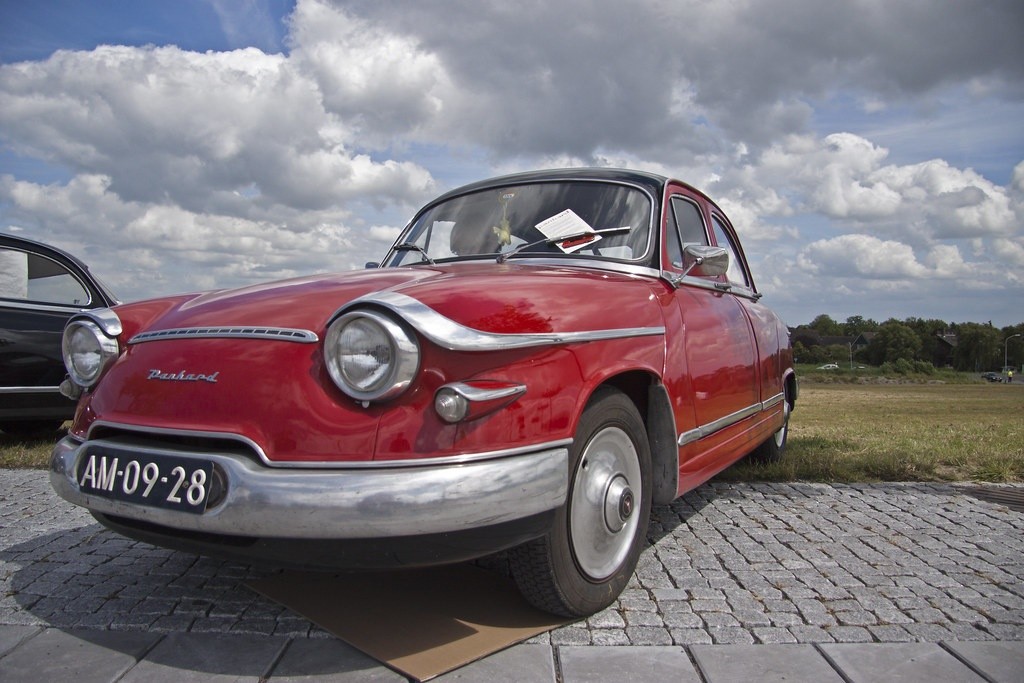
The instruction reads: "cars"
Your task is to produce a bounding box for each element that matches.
[982,371,1002,382]
[45,165,800,619]
[817,364,840,370]
[1,233,122,433]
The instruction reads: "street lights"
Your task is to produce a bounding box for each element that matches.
[1003,333,1020,382]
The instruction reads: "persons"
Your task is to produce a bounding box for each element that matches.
[450,214,502,255]
[1007,368,1012,382]
